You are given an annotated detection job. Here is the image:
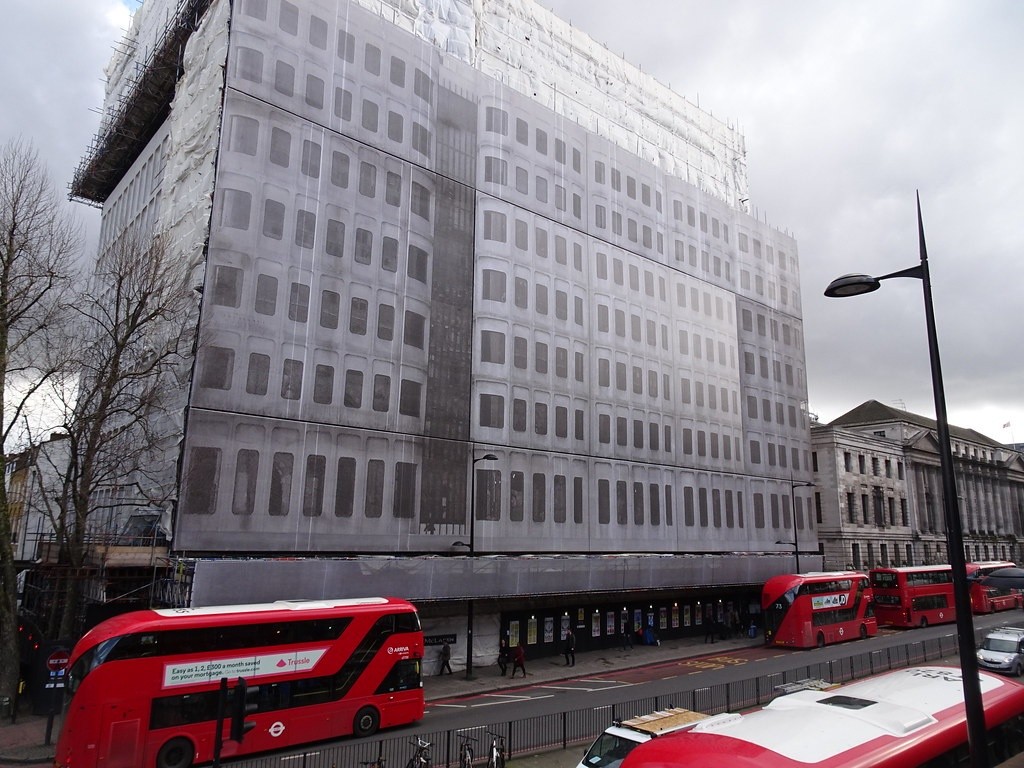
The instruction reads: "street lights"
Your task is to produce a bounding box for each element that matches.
[451,437,498,678]
[822,189,992,768]
[774,474,817,575]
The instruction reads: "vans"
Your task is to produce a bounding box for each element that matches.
[577,704,711,768]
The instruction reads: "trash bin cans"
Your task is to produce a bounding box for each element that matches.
[749,626,757,639]
[0,696,12,718]
[640,626,653,645]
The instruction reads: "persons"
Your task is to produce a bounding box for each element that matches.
[562,628,576,668]
[705,605,760,644]
[437,639,453,676]
[509,643,528,679]
[497,638,509,678]
[623,618,661,652]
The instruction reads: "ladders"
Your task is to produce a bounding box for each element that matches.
[774,678,823,694]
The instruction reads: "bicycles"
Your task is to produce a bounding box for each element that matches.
[485,731,506,768]
[405,734,435,768]
[457,734,479,768]
[360,757,386,768]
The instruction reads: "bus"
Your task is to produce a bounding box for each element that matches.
[609,665,1024,768]
[868,564,974,625]
[964,561,1024,614]
[761,570,879,650]
[54,596,430,768]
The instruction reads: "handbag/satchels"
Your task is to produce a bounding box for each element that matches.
[442,654,449,661]
[498,656,508,663]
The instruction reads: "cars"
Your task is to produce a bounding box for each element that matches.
[977,626,1024,678]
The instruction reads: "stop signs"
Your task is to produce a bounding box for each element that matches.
[46,650,71,672]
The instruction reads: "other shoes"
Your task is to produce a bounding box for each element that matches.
[500,672,506,676]
[523,674,526,678]
[447,673,453,675]
[510,675,514,679]
[437,674,443,676]
[570,664,575,667]
[564,663,570,666]
